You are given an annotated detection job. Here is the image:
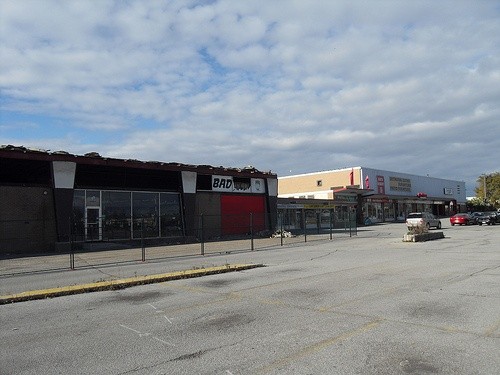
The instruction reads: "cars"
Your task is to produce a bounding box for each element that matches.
[450,213,479,226]
[472,211,485,219]
[406,212,441,231]
[476,211,500,225]
